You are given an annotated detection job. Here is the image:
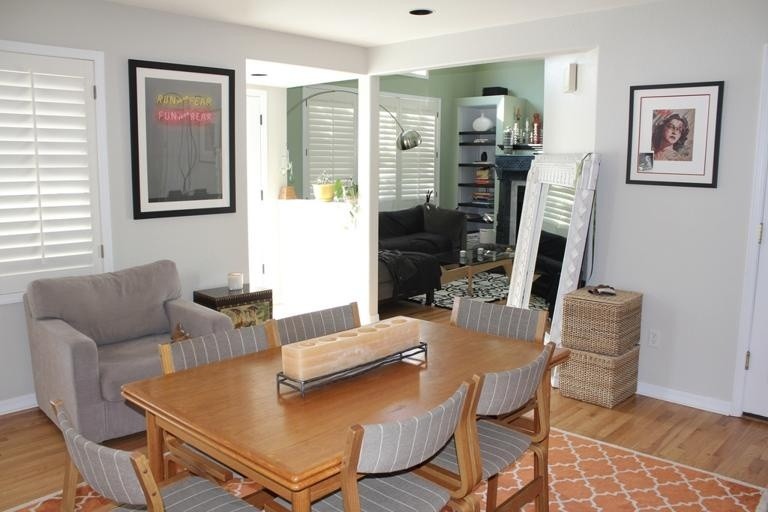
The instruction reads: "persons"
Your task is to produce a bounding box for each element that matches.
[653,114,690,159]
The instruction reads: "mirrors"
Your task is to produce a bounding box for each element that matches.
[508,152,600,387]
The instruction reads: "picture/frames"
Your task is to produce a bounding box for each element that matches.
[129,59,235,220]
[624,81,725,188]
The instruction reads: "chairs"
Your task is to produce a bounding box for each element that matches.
[447,298,548,343]
[158,320,273,482]
[269,303,363,347]
[422,341,555,511]
[274,377,474,511]
[45,400,261,511]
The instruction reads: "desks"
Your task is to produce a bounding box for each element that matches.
[195,286,273,326]
[120,315,566,511]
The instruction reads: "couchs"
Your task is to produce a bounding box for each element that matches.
[379,205,466,304]
[536,226,569,293]
[21,259,235,443]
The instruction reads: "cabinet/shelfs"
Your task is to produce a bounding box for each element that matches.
[455,95,525,248]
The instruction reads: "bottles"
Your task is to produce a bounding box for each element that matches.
[523,118,532,144]
[513,123,523,144]
[503,125,514,154]
[532,113,541,143]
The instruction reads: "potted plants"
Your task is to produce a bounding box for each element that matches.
[312,175,335,201]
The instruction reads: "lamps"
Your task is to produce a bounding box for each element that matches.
[287,89,423,149]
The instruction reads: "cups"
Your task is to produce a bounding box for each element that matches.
[476,248,484,261]
[227,271,244,290]
[460,249,467,265]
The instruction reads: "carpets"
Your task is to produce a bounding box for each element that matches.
[0,424,768,511]
[528,292,550,310]
[403,271,509,311]
